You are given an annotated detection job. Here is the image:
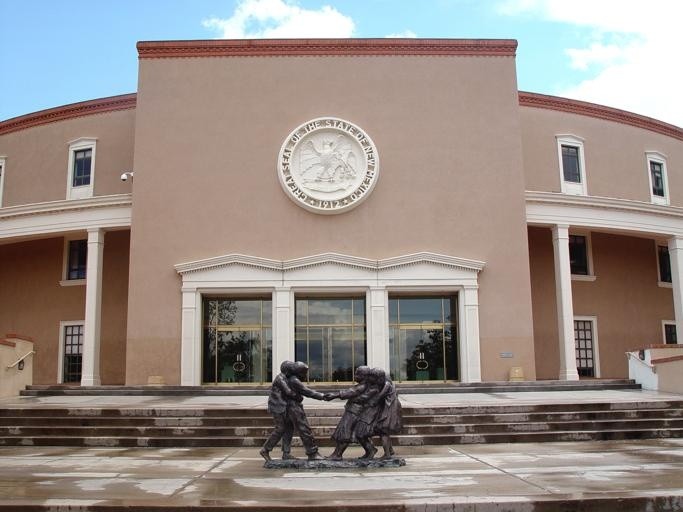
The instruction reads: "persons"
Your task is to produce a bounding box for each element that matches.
[285,360,330,459]
[364,376,400,461]
[259,359,303,462]
[323,365,370,461]
[349,366,386,460]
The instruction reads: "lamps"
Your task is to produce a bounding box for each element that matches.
[120,171,134,181]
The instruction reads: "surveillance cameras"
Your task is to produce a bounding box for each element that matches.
[121,175,127,181]
